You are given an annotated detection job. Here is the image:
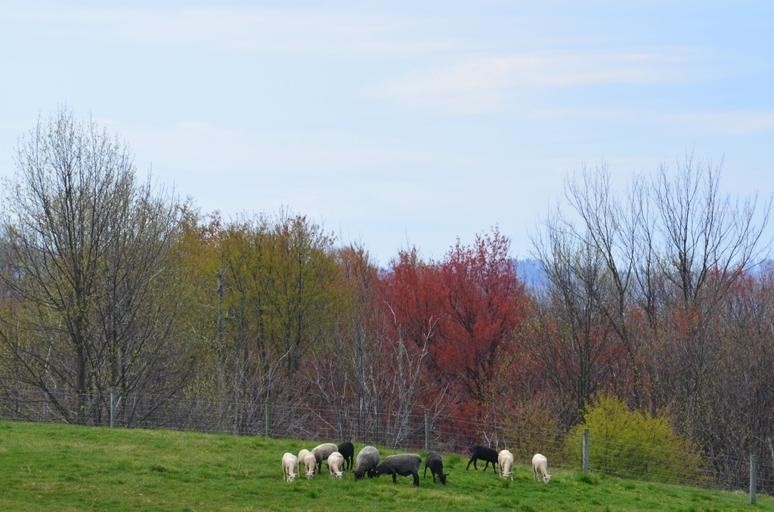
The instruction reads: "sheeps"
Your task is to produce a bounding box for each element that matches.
[351,446,381,482]
[423,452,449,485]
[281,452,297,485]
[297,441,354,481]
[532,453,552,486]
[498,449,514,483]
[465,444,499,474]
[372,454,422,487]
